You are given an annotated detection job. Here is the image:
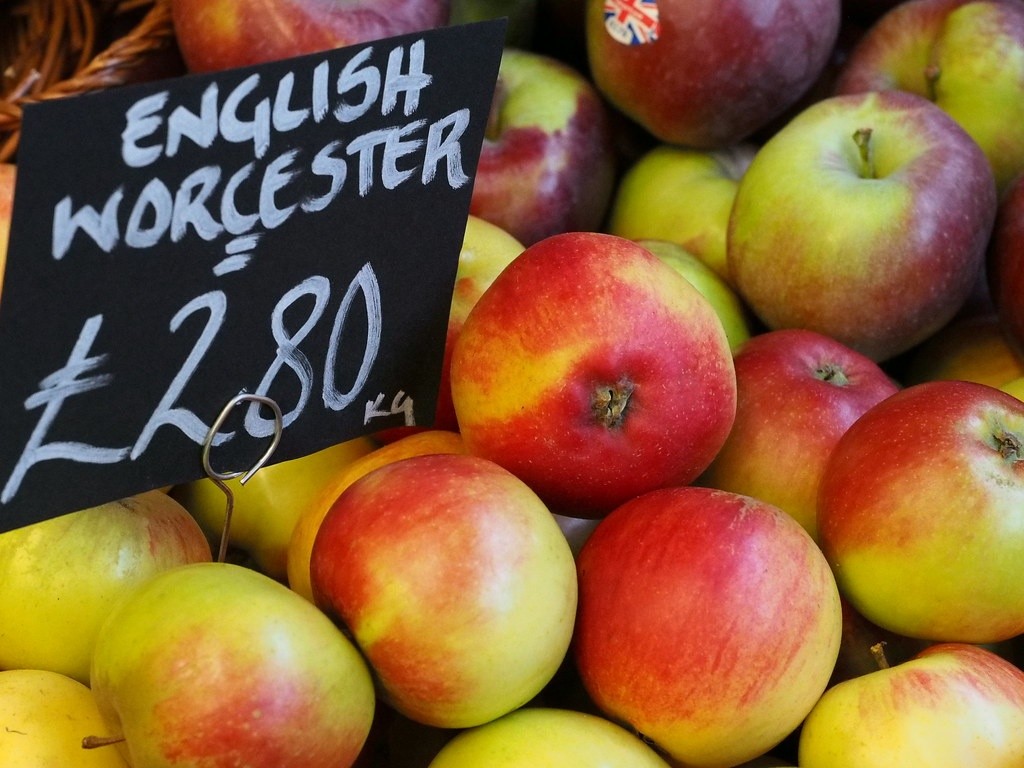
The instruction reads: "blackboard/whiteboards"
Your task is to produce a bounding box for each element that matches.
[0,16,509,534]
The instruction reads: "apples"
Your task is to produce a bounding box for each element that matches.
[0,0,1024,768]
